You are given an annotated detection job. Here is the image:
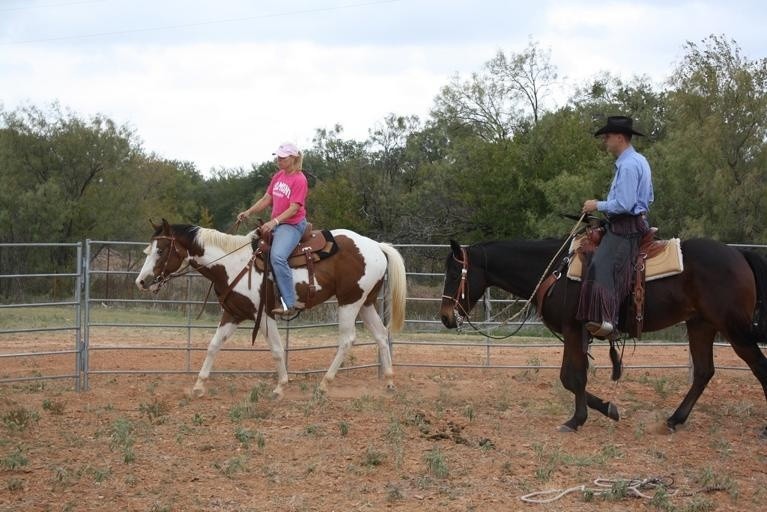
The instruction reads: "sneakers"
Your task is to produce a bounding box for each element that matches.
[271,304,295,315]
[585,320,619,341]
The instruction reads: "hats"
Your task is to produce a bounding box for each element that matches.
[594,116,646,137]
[272,143,299,158]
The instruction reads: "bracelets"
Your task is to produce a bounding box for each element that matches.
[272,218,279,226]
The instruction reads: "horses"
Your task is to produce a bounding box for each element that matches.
[440,237,767,435]
[134,217,407,405]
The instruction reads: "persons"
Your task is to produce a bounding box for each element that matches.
[236,142,308,319]
[579,111,657,343]
